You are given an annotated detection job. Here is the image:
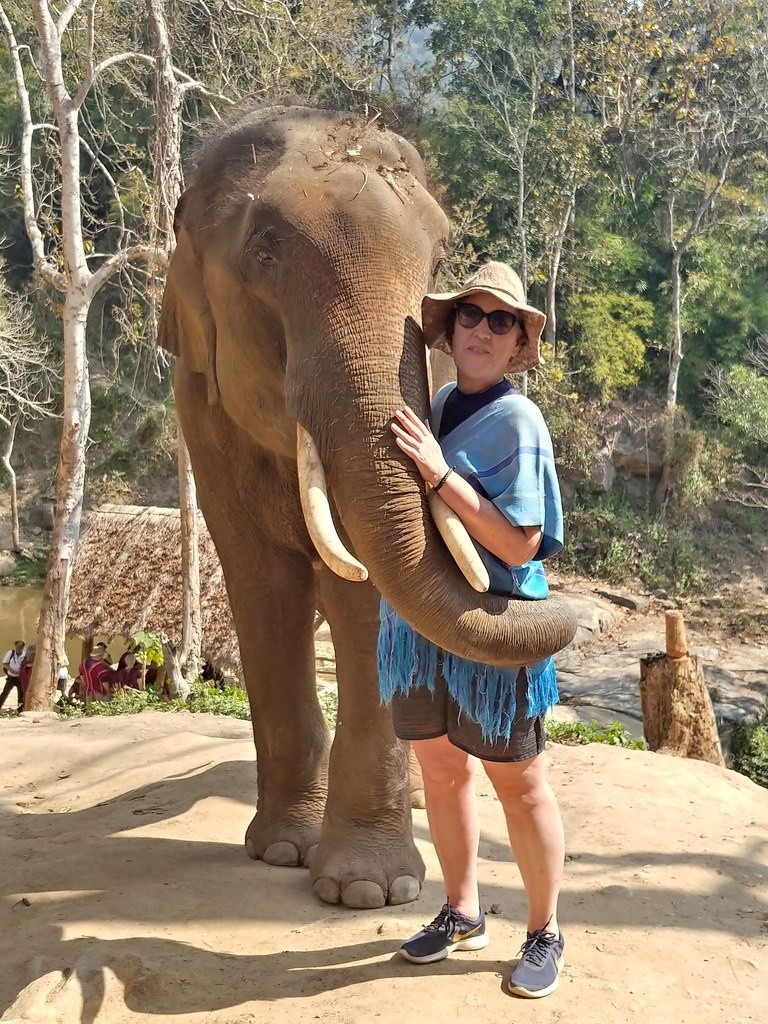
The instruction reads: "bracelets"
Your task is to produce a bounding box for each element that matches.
[434,466,454,493]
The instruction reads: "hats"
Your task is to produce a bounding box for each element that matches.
[422,261,546,372]
[90,646,109,658]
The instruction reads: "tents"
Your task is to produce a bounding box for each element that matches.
[64,503,247,694]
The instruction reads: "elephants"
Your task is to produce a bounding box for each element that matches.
[152,94,578,911]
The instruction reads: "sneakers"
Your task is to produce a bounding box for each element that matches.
[401,896,488,962]
[507,913,565,999]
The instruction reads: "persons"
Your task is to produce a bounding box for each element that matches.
[376,259,566,999]
[0,640,159,713]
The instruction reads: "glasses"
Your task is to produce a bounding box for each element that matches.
[453,300,525,336]
